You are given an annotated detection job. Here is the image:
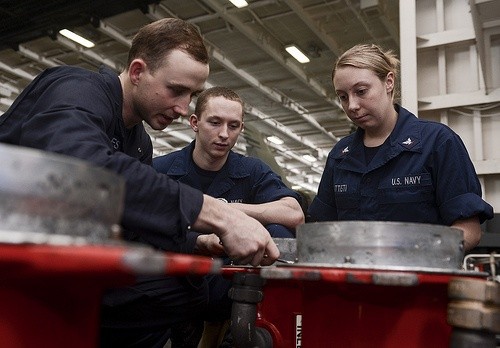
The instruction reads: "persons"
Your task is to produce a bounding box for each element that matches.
[150,86,304,348]
[0,16,280,348]
[304,42,494,250]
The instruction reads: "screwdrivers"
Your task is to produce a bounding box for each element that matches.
[219,239,299,267]
